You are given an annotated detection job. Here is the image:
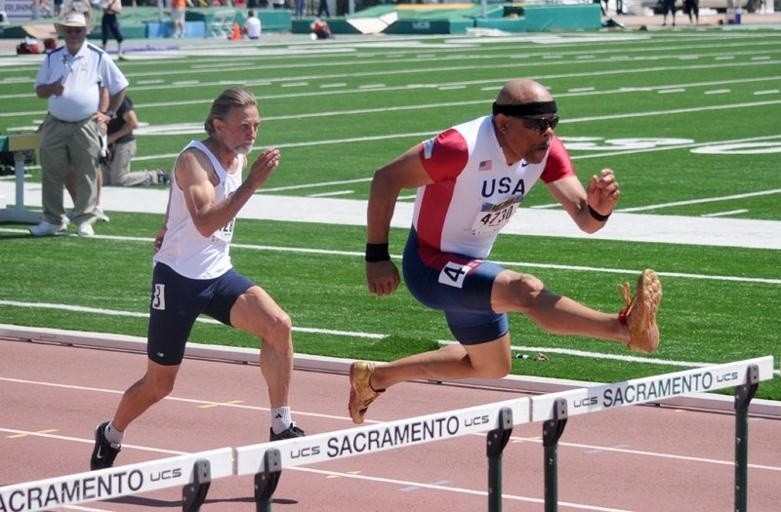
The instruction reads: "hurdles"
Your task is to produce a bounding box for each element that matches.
[0,354,775,512]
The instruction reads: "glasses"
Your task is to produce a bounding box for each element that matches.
[523,116,559,129]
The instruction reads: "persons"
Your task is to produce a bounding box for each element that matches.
[295,0,305,19]
[89,88,305,469]
[241,10,261,40]
[29,11,129,235]
[72,0,94,38]
[593,0,764,27]
[101,0,123,57]
[311,19,329,39]
[348,78,660,423]
[100,94,171,187]
[317,0,330,18]
[40,0,63,18]
[171,0,193,39]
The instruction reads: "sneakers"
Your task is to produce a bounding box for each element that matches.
[91,421,121,470]
[349,361,378,424]
[29,221,94,237]
[618,269,662,351]
[270,423,305,441]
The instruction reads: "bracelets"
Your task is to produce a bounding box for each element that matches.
[365,244,391,262]
[106,111,119,122]
[587,205,611,221]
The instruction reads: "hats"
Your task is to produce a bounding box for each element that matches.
[54,12,90,32]
